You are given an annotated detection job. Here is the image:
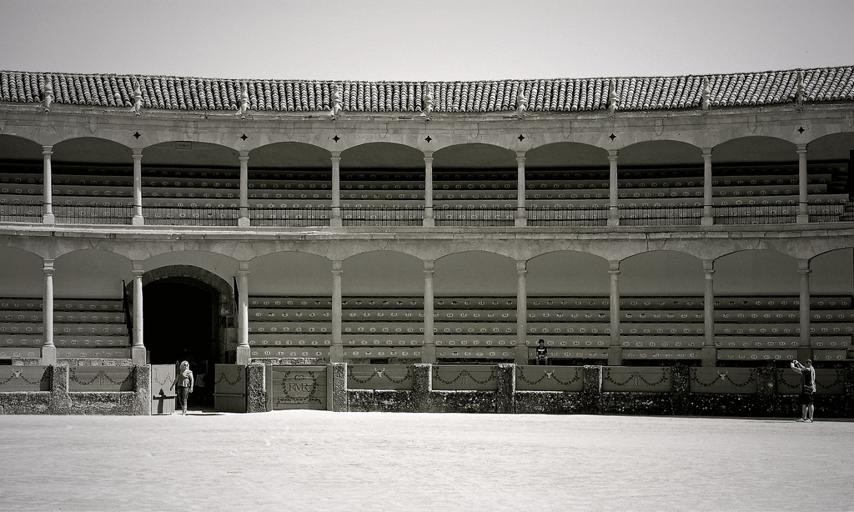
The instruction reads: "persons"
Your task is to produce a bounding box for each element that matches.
[799,359,817,423]
[791,359,813,423]
[173,360,180,375]
[170,360,194,415]
[535,338,547,365]
